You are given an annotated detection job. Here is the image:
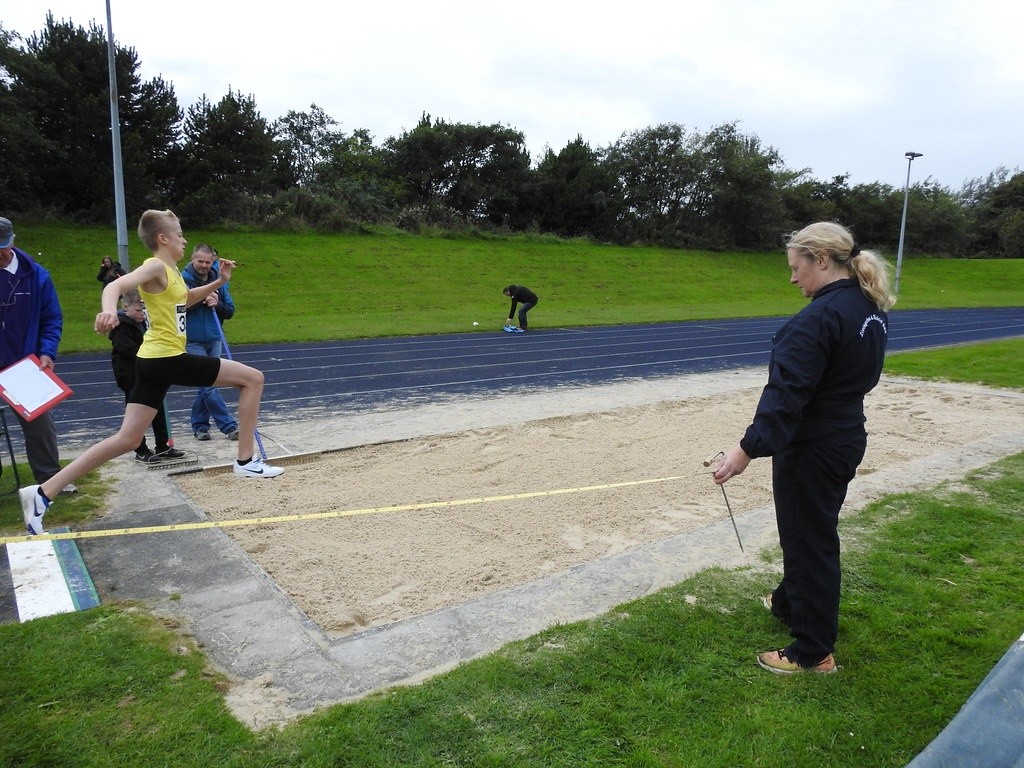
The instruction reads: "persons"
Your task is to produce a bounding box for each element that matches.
[713,221,900,675]
[0,216,80,494]
[502,284,539,332]
[107,289,185,463]
[18,208,284,536]
[97,256,127,301]
[180,243,240,442]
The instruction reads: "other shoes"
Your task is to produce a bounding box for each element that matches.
[58,483,79,496]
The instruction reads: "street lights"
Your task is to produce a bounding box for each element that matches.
[894,151,923,294]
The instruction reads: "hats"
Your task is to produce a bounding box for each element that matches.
[0,217,17,249]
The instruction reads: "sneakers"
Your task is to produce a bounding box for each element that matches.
[226,428,239,441]
[194,429,211,440]
[762,592,773,610]
[233,453,285,479]
[17,483,53,536]
[133,449,162,464]
[755,647,839,677]
[154,446,186,458]
